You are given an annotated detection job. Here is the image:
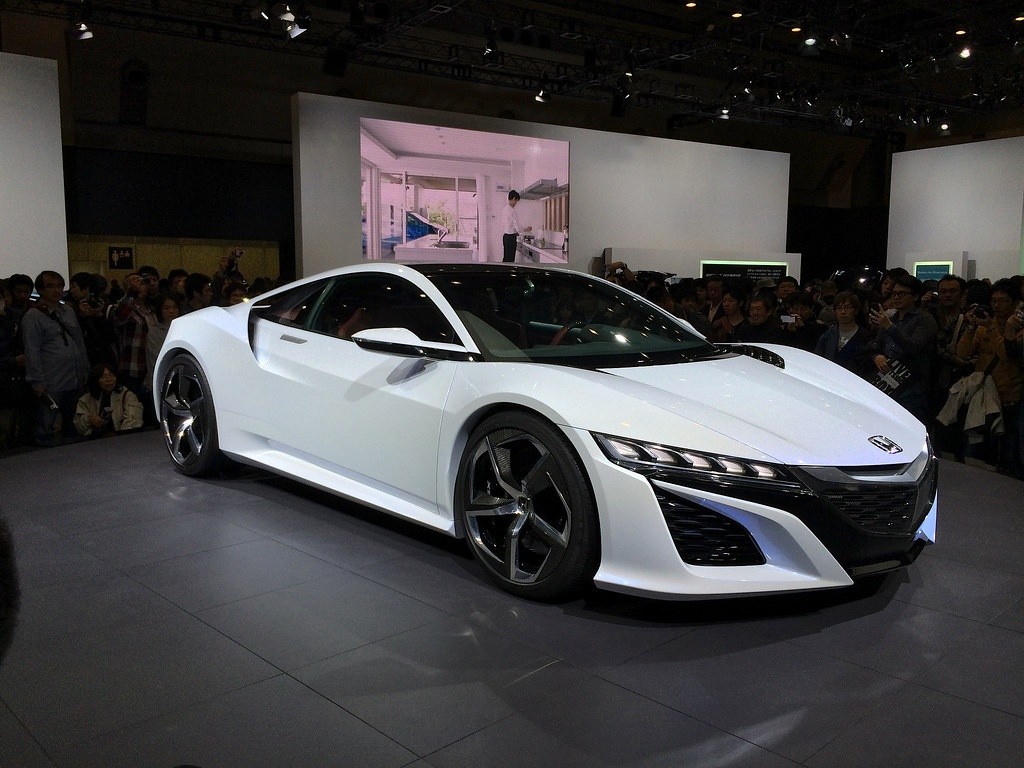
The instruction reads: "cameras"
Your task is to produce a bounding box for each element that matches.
[84,290,101,309]
[928,290,941,304]
[1017,312,1024,320]
[224,249,243,275]
[974,305,994,319]
[140,273,150,289]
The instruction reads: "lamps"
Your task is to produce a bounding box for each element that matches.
[534,89,549,103]
[284,20,307,39]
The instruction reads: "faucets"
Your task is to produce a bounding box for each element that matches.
[435,228,451,245]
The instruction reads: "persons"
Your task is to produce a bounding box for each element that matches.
[0,256,292,449]
[570,261,1024,481]
[502,190,533,263]
[0,517,21,663]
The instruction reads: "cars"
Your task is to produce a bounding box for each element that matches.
[362,204,448,255]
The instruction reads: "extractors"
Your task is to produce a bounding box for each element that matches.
[517,179,568,202]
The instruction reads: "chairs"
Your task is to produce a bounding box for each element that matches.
[458,289,525,348]
[371,284,433,342]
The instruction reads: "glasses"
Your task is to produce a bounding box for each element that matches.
[837,305,858,311]
[891,291,914,297]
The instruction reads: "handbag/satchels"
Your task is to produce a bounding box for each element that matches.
[939,358,974,388]
[873,359,913,396]
[957,397,995,433]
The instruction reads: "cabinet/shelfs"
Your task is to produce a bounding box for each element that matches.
[515,242,558,264]
[541,192,569,246]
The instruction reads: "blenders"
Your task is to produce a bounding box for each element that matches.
[561,224,568,253]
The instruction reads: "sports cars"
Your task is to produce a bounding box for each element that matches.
[152,260,940,604]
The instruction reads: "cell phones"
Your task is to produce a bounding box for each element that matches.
[781,316,796,323]
[870,302,879,325]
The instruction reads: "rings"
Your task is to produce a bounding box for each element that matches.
[878,314,880,317]
[879,361,882,363]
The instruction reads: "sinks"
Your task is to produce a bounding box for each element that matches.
[430,240,470,249]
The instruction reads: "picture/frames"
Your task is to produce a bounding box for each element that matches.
[106,246,137,272]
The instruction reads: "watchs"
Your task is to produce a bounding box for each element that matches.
[621,264,627,270]
